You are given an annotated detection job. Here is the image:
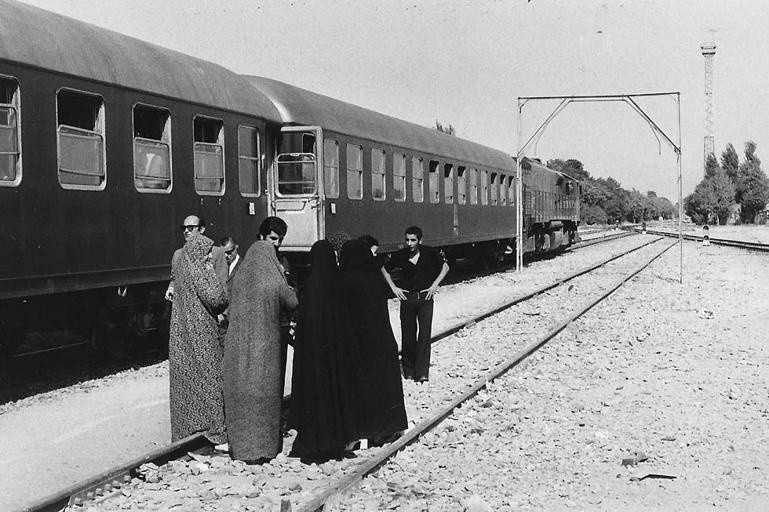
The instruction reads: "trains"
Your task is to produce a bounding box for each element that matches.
[0,1,583,387]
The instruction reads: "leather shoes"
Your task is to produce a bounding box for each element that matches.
[408,375,428,381]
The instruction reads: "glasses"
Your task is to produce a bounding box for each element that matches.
[182,225,200,231]
[225,248,235,254]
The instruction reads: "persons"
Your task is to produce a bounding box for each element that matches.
[381,225,451,383]
